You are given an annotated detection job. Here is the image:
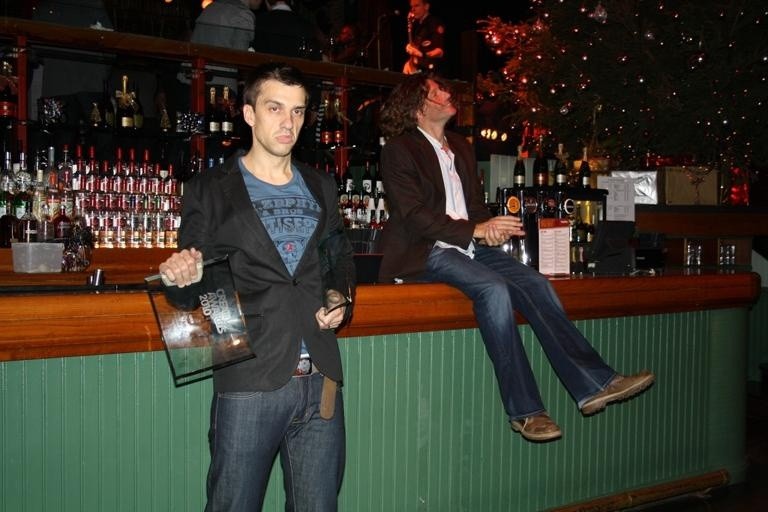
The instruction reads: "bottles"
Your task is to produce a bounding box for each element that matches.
[1,77,603,278]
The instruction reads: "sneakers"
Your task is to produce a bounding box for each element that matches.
[511,411,562,441]
[582,370,656,415]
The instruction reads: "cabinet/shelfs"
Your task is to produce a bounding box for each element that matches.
[0,20,476,290]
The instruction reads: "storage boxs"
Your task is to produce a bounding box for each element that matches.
[658,165,718,205]
[10,242,65,273]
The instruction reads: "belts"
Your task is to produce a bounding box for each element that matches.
[291,353,337,421]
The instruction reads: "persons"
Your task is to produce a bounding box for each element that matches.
[377,71,654,442]
[254,2,321,57]
[402,0,444,70]
[159,65,357,512]
[192,0,262,51]
[330,24,360,63]
